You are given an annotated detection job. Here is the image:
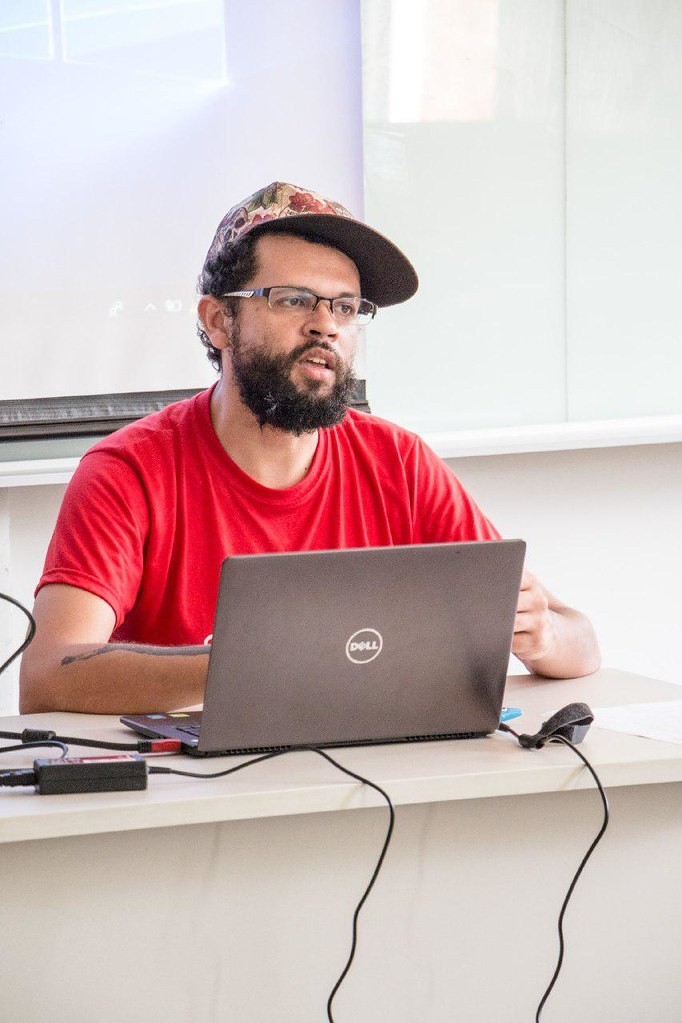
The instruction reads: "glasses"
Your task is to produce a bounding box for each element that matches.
[221,285,378,326]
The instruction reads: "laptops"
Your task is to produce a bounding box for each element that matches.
[118,539,527,760]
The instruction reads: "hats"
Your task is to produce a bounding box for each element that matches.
[203,181,419,308]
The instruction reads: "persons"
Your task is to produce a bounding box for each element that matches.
[19,182,602,714]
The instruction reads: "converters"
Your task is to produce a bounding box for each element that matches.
[36,756,147,795]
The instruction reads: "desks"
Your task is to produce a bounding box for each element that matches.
[0,666,681,1023]
